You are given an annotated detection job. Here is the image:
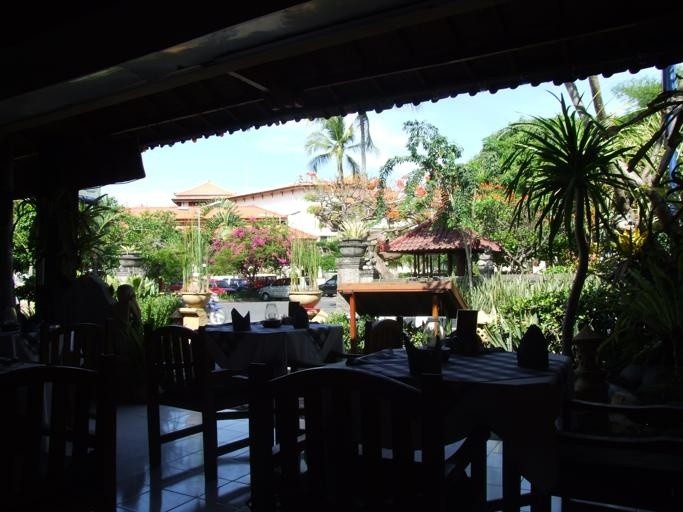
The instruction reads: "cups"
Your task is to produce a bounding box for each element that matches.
[265,304,277,320]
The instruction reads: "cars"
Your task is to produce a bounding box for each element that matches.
[158,272,337,301]
[474,254,547,274]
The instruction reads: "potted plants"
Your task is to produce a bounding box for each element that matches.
[337,218,368,257]
[119,244,144,267]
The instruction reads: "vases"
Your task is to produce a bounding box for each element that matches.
[288,291,322,308]
[181,292,212,308]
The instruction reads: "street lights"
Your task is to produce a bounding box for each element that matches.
[196,200,224,289]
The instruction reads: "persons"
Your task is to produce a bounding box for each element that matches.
[107,285,143,394]
